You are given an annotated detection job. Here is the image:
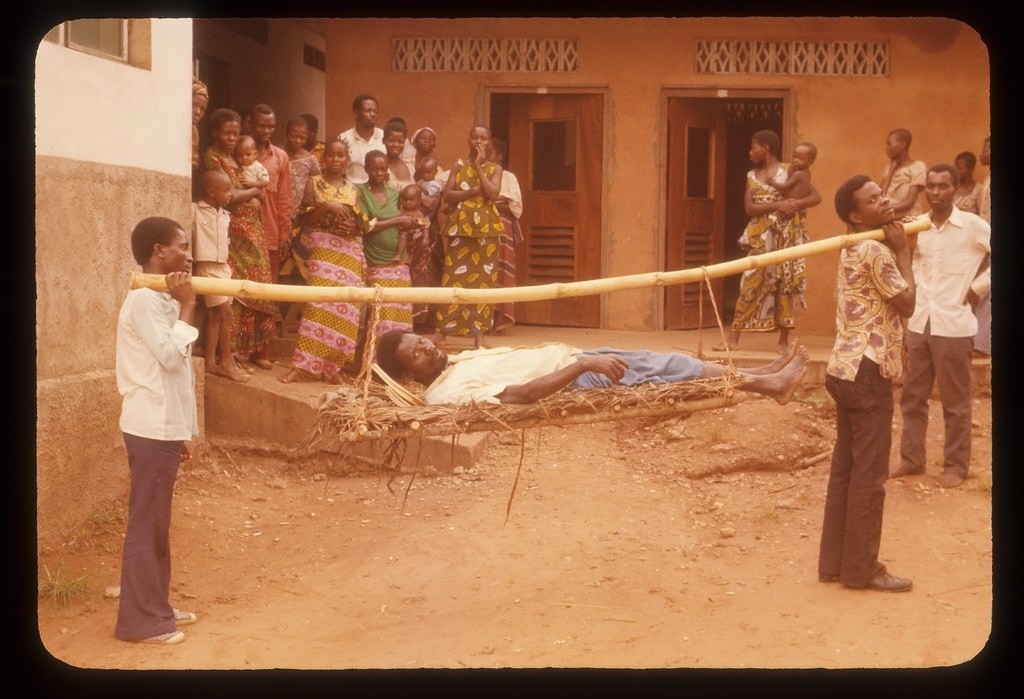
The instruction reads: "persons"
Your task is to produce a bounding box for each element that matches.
[971,135,992,358]
[377,327,808,406]
[820,175,918,594]
[952,151,982,212]
[880,128,927,222]
[767,142,818,246]
[117,218,199,644]
[712,130,822,355]
[187,77,523,383]
[888,163,992,487]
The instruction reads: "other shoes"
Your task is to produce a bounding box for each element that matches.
[866,571,912,591]
[820,570,842,582]
[142,631,186,644]
[171,607,196,625]
